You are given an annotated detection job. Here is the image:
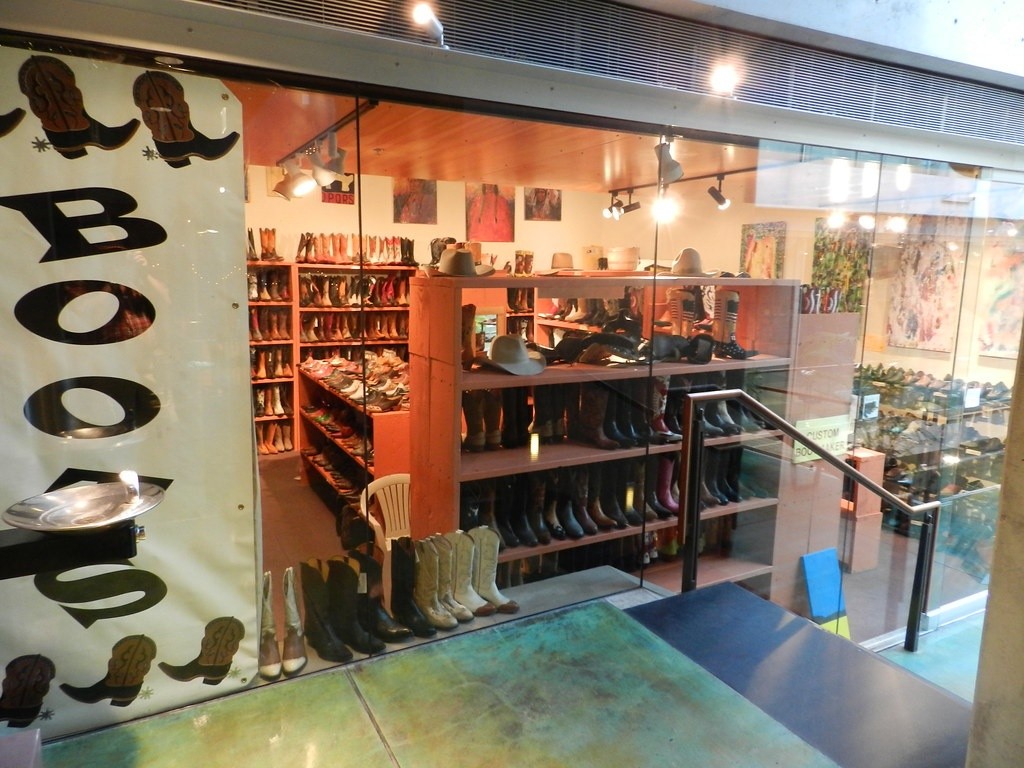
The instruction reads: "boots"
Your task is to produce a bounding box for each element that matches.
[391,536,437,637]
[430,532,473,622]
[469,528,519,615]
[258,571,282,680]
[282,566,308,676]
[450,530,495,616]
[415,537,457,629]
[347,541,414,643]
[461,285,779,590]
[325,556,386,653]
[244,226,419,455]
[300,558,354,663]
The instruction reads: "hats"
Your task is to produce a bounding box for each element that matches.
[474,335,546,376]
[423,248,496,277]
[534,252,584,276]
[657,247,719,277]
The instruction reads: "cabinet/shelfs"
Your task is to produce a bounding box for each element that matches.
[247,261,298,458]
[298,264,420,451]
[533,269,670,349]
[839,446,882,573]
[407,275,801,588]
[298,367,410,535]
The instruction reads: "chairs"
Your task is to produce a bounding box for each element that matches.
[359,473,410,618]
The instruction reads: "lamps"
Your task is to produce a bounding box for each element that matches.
[612,188,641,220]
[654,138,684,186]
[602,192,623,219]
[283,155,316,197]
[272,169,293,202]
[304,138,335,186]
[413,4,451,52]
[326,133,348,179]
[708,176,731,211]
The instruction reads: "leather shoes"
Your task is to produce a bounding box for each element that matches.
[851,361,1011,583]
